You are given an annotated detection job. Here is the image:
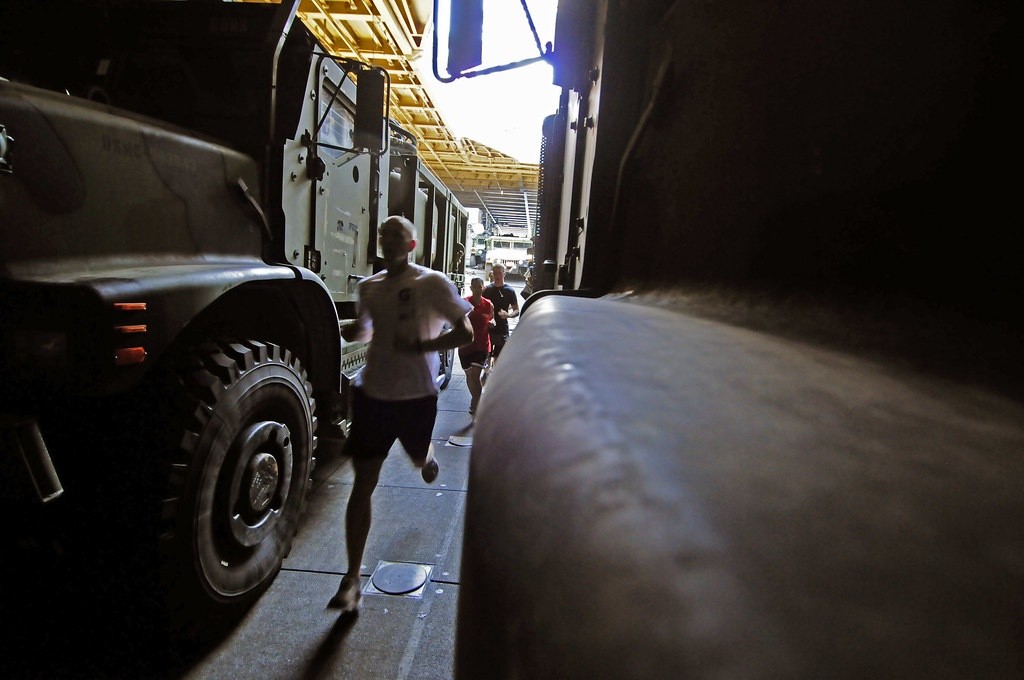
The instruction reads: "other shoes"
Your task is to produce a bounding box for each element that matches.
[483,359,490,369]
[469,400,475,413]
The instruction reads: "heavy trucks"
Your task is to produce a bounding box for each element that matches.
[0,0,471,617]
[424,3,1024,680]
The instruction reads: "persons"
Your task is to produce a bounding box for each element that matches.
[324,216,477,612]
[453,276,498,417]
[478,263,520,386]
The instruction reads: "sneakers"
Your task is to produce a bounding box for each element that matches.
[420,459,439,483]
[327,579,364,611]
